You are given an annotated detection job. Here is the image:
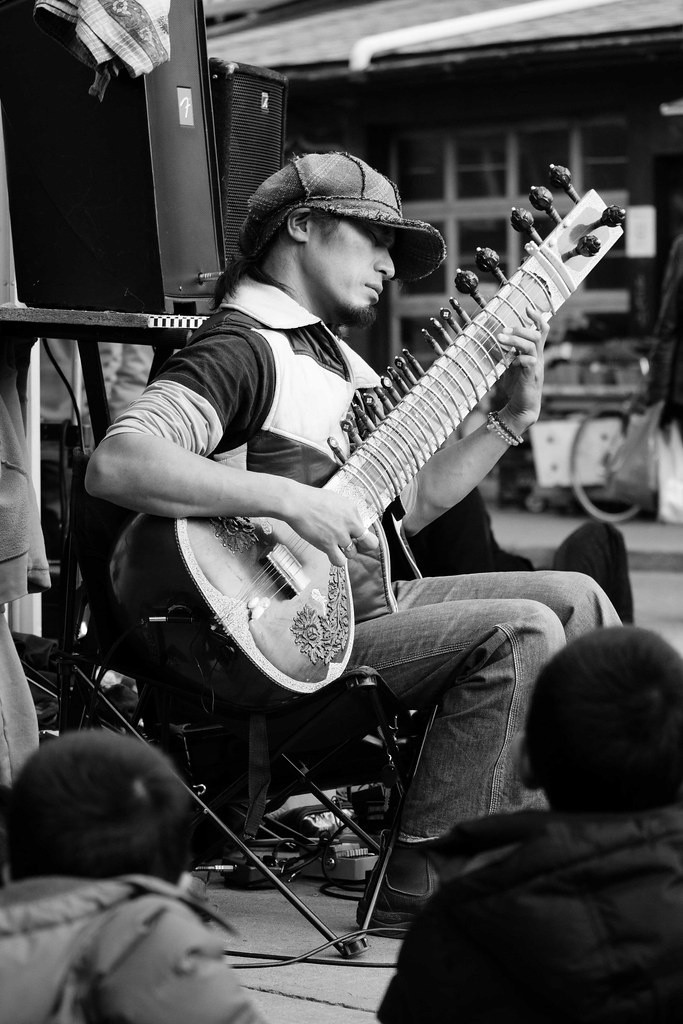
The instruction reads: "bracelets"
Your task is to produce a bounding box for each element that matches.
[487,411,524,448]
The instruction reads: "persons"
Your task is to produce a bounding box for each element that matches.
[630,185,683,443]
[0,625,682,1024]
[83,147,622,940]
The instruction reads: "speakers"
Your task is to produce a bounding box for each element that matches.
[207,56,289,270]
[0,0,226,319]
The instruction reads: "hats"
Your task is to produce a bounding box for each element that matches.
[238,151,448,282]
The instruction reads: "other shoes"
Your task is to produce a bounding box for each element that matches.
[357,828,442,939]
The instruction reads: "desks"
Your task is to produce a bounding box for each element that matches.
[0,306,212,450]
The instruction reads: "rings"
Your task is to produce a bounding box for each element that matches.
[351,528,369,544]
[339,541,355,553]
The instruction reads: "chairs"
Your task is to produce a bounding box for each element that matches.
[59,420,442,959]
